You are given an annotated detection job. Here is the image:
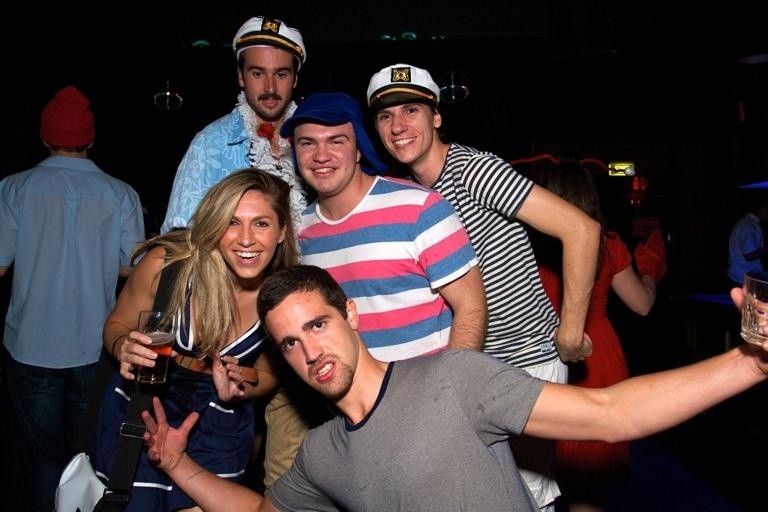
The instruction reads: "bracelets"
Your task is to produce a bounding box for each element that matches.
[111,333,127,358]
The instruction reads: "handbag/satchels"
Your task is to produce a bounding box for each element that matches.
[56,453,129,512]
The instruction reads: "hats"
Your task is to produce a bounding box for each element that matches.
[366,63,441,113]
[232,17,305,63]
[281,92,386,176]
[41,88,93,147]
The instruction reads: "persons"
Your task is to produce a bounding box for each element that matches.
[94,165,300,511]
[157,15,318,242]
[141,261,768,511]
[0,83,150,512]
[511,152,668,474]
[363,60,608,511]
[260,89,489,494]
[725,198,767,292]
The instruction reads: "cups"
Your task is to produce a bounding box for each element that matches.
[739,270,768,346]
[137,311,176,386]
